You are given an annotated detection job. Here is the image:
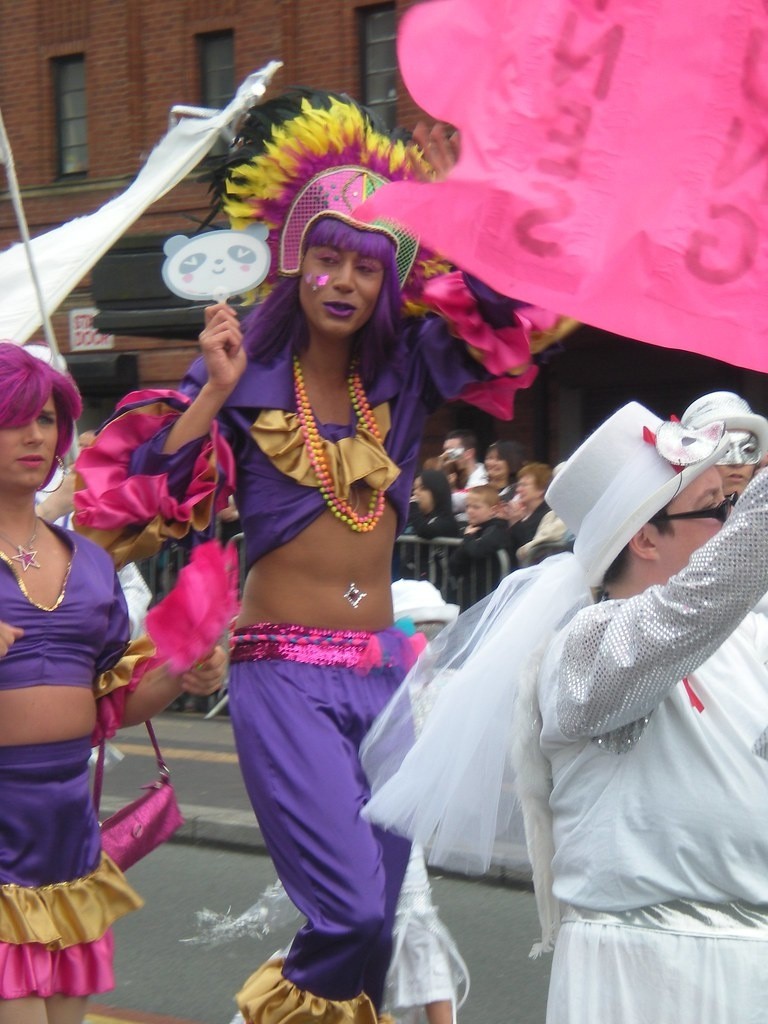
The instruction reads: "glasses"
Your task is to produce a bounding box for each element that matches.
[659,490,742,527]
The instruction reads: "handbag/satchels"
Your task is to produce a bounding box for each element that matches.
[90,716,186,876]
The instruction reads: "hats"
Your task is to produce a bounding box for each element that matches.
[678,391,768,446]
[544,399,730,589]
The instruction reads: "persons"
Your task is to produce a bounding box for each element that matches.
[380,390,767,1022]
[66,86,581,1024]
[0,341,228,1024]
[32,431,242,717]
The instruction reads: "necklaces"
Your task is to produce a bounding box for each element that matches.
[293,341,387,533]
[0,514,41,571]
